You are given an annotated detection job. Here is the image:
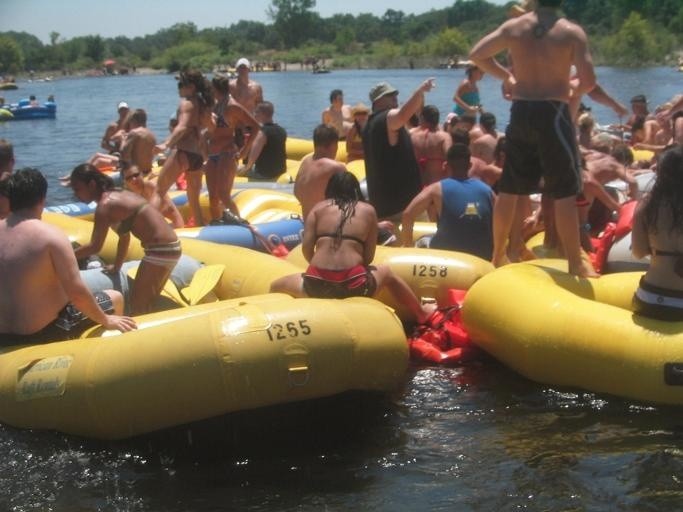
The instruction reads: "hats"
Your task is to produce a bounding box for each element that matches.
[118,102,128,109]
[369,82,398,102]
[236,57,250,68]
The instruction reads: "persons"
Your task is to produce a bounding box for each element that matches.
[100,57,288,227]
[524,94,683,321]
[502,1,629,264]
[293,61,533,268]
[2,76,16,89]
[271,171,438,325]
[464,0,603,279]
[69,163,182,317]
[0,94,55,120]
[1,138,137,349]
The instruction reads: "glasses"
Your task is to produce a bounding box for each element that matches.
[126,173,139,181]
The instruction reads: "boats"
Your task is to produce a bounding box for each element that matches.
[311,68,330,74]
[282,217,566,317]
[212,66,239,80]
[523,142,662,265]
[76,186,309,258]
[0,82,19,90]
[0,98,60,120]
[678,67,682,72]
[229,137,368,185]
[456,249,683,405]
[0,209,412,443]
